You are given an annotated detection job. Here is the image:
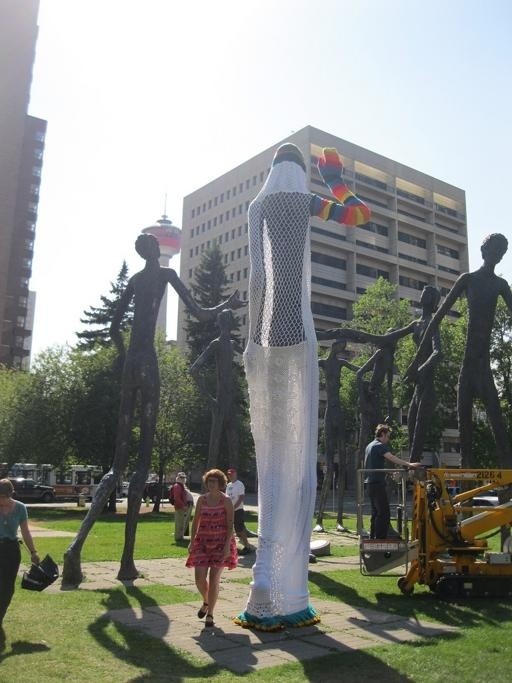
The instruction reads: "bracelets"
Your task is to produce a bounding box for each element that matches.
[31,550,37,554]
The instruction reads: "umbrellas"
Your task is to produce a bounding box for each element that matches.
[19,539,59,592]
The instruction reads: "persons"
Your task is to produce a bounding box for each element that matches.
[328,284,442,468]
[362,423,420,539]
[172,471,194,543]
[63,233,249,584]
[312,340,362,532]
[405,233,512,551]
[184,469,238,626]
[0,479,41,650]
[354,326,398,534]
[190,308,245,495]
[225,467,251,556]
[241,142,372,628]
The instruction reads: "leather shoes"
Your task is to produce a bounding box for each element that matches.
[198,602,213,626]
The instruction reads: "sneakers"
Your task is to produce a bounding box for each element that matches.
[240,546,250,554]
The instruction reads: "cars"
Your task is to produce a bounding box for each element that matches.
[143,482,173,503]
[10,478,56,503]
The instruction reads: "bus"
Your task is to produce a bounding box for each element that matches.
[0,462,105,502]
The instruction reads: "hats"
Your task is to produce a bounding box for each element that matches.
[227,469,236,474]
[176,472,187,478]
